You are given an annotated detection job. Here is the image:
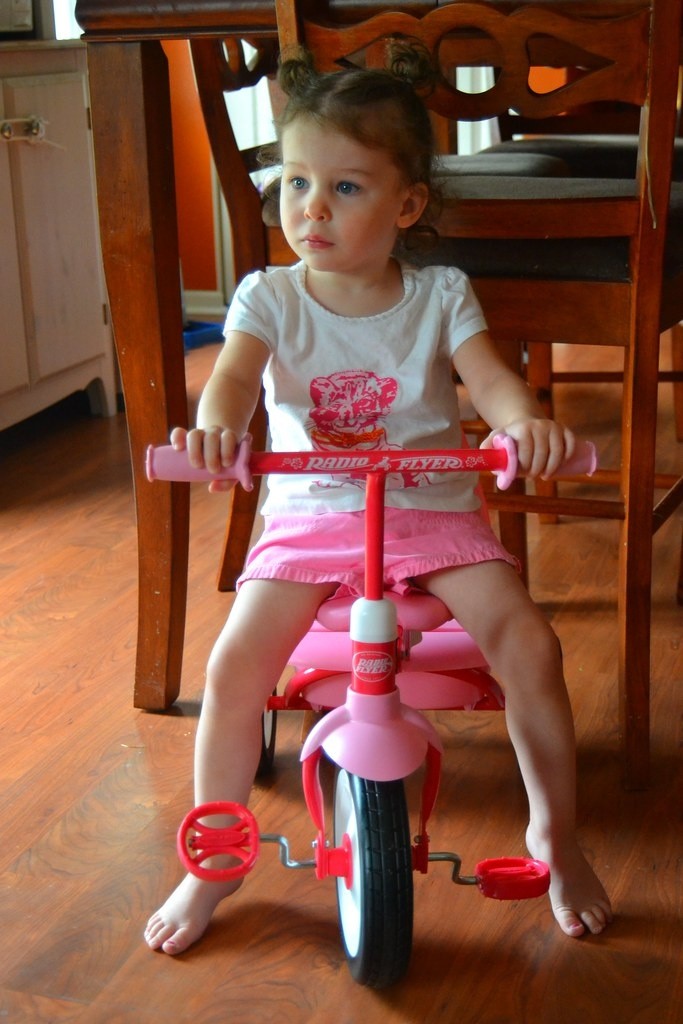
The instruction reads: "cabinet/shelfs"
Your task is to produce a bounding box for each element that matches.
[0,50,116,442]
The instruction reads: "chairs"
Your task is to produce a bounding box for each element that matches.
[184,0,682,791]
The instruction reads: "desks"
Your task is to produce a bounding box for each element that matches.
[74,1,683,712]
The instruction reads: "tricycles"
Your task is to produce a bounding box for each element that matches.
[148,434,598,986]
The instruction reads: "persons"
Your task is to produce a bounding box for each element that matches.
[142,33,616,957]
